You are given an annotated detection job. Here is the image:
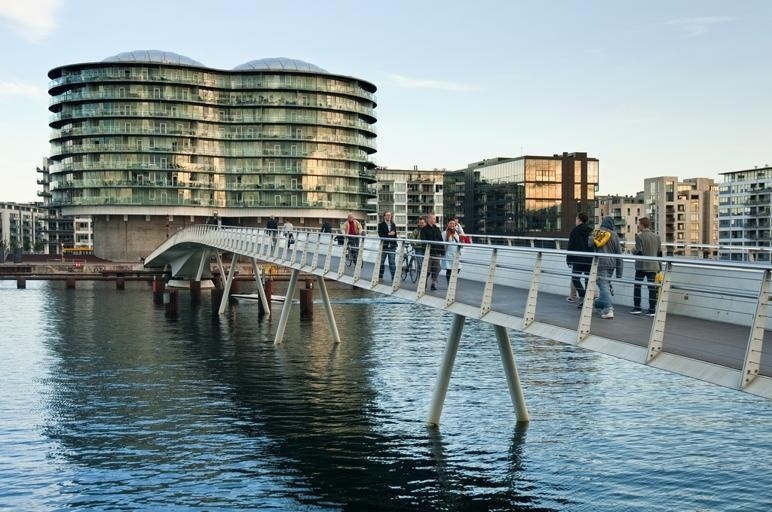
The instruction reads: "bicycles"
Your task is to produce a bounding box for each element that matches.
[397,241,420,284]
[341,233,364,267]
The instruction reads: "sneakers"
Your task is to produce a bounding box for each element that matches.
[601,310,615,319]
[645,310,654,317]
[629,307,643,314]
[566,297,584,308]
[431,285,437,290]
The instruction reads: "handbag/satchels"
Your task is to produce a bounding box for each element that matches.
[655,272,664,303]
[290,240,295,244]
[591,228,611,247]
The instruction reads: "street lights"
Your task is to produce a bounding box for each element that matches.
[61,241,64,263]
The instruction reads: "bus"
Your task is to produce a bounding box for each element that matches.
[61,246,96,256]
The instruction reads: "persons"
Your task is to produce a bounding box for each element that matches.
[441,218,464,286]
[567,211,595,309]
[409,217,428,277]
[320,221,332,233]
[421,213,445,291]
[588,216,623,319]
[566,268,598,302]
[266,214,278,251]
[333,232,344,245]
[379,210,397,281]
[630,218,662,317]
[454,216,464,235]
[282,218,294,249]
[343,214,362,265]
